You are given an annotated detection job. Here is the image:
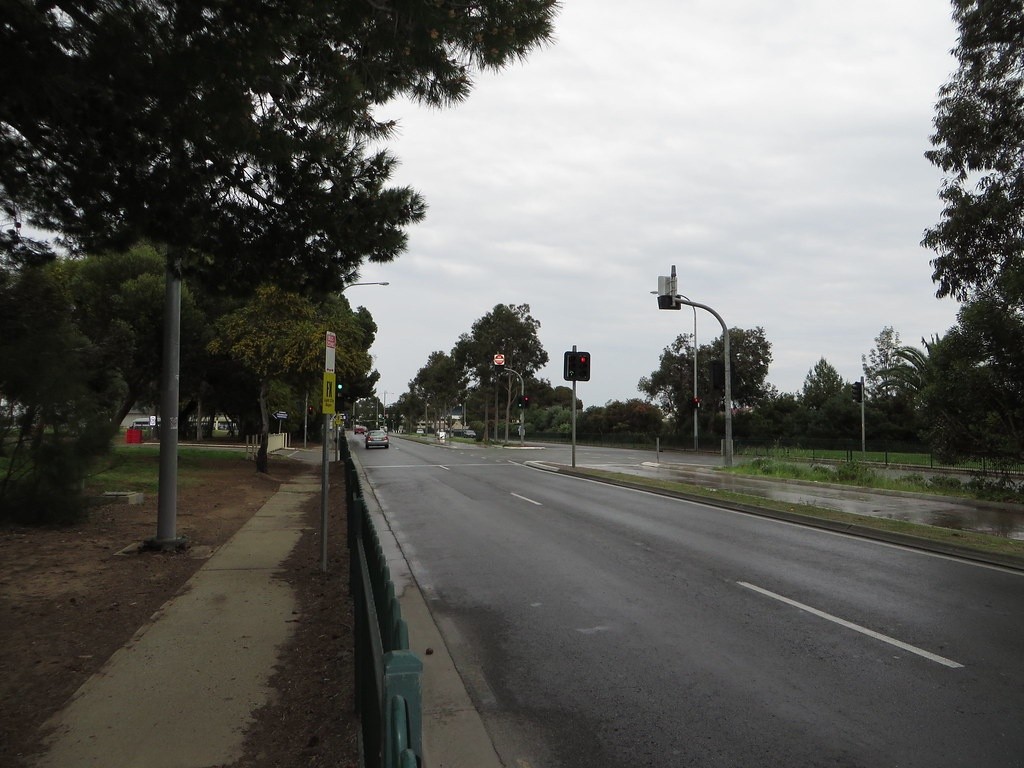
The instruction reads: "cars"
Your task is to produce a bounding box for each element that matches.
[380,426,387,432]
[354,428,365,434]
[464,430,476,438]
[365,430,389,449]
[417,429,424,434]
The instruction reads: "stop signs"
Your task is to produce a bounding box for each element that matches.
[494,354,504,365]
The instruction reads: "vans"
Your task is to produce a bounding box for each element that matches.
[435,428,454,438]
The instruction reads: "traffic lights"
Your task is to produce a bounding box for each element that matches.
[308,406,313,415]
[852,382,862,403]
[691,399,699,407]
[517,396,529,408]
[334,383,344,414]
[564,352,590,381]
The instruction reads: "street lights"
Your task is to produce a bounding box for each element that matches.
[376,392,394,425]
[650,291,698,451]
[504,368,524,447]
